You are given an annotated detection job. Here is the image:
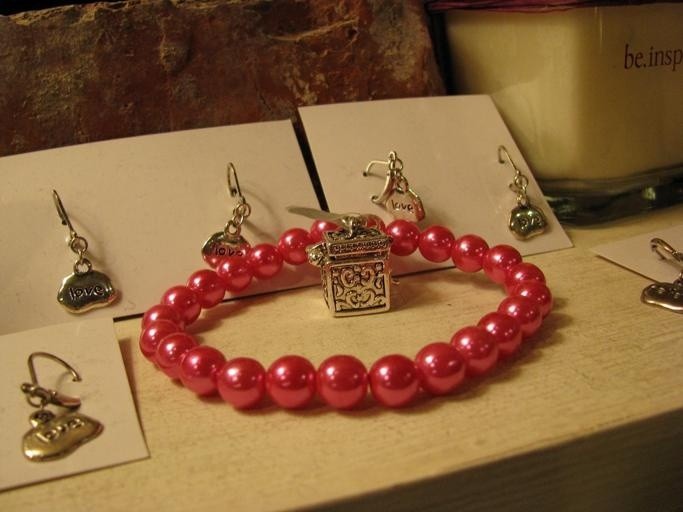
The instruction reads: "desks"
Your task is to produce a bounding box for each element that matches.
[0,206,683,512]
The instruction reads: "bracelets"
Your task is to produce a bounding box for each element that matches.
[139,214,552,412]
